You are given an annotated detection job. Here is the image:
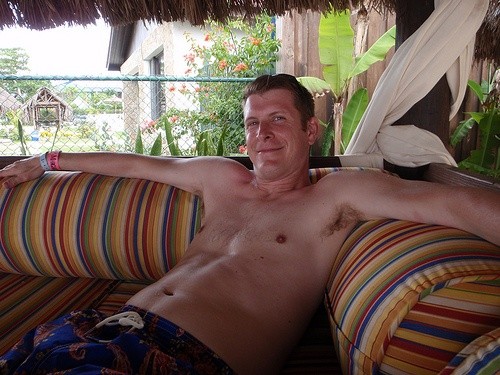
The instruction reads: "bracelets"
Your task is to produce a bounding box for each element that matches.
[39,151,50,171]
[47,151,62,171]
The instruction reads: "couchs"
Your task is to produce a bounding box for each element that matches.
[0,156,500,375]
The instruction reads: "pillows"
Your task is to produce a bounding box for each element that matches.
[324,219,500,375]
[0,167,400,283]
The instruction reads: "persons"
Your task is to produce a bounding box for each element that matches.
[0,70,499,375]
